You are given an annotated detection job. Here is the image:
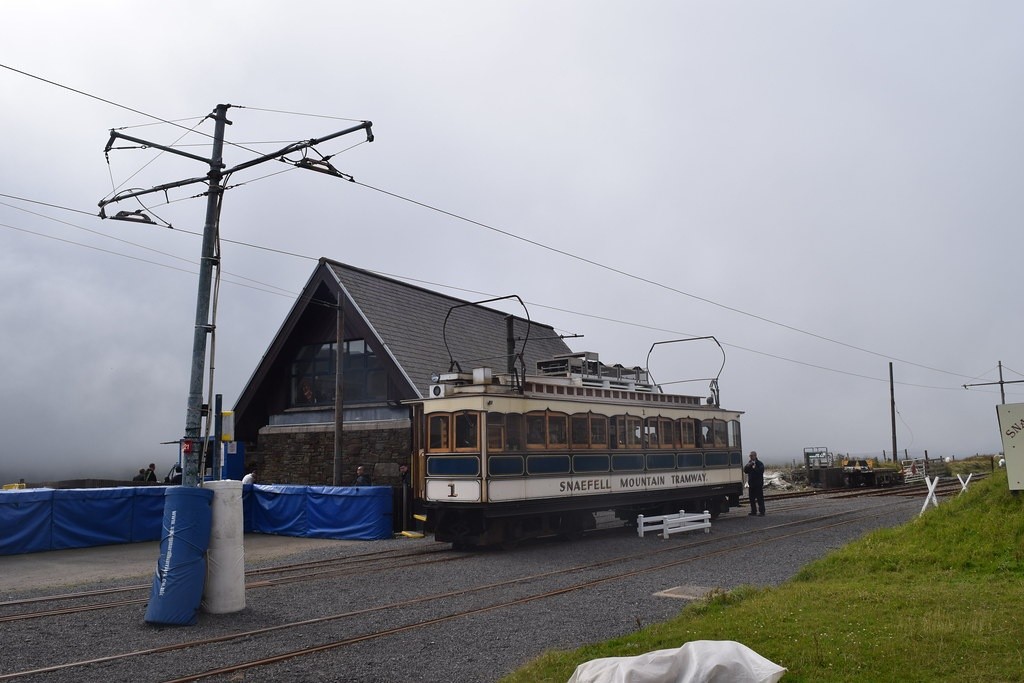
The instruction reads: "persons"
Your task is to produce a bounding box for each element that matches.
[241,469,259,485]
[399,463,413,521]
[353,466,371,487]
[998,455,1005,468]
[744,451,766,517]
[132,463,182,484]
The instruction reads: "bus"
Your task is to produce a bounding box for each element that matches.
[399,351,746,552]
